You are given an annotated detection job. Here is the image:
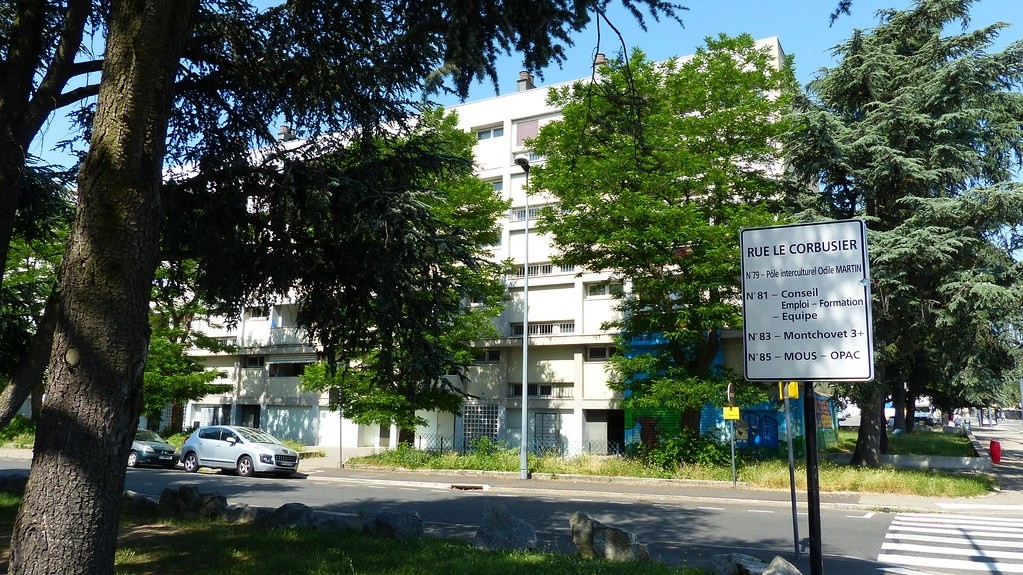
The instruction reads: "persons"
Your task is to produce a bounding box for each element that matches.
[949,412,962,427]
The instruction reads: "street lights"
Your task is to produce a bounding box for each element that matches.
[514,157,531,482]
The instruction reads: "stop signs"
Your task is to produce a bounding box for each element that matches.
[727,383,735,407]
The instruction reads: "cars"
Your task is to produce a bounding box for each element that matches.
[177,424,300,478]
[125,427,178,472]
